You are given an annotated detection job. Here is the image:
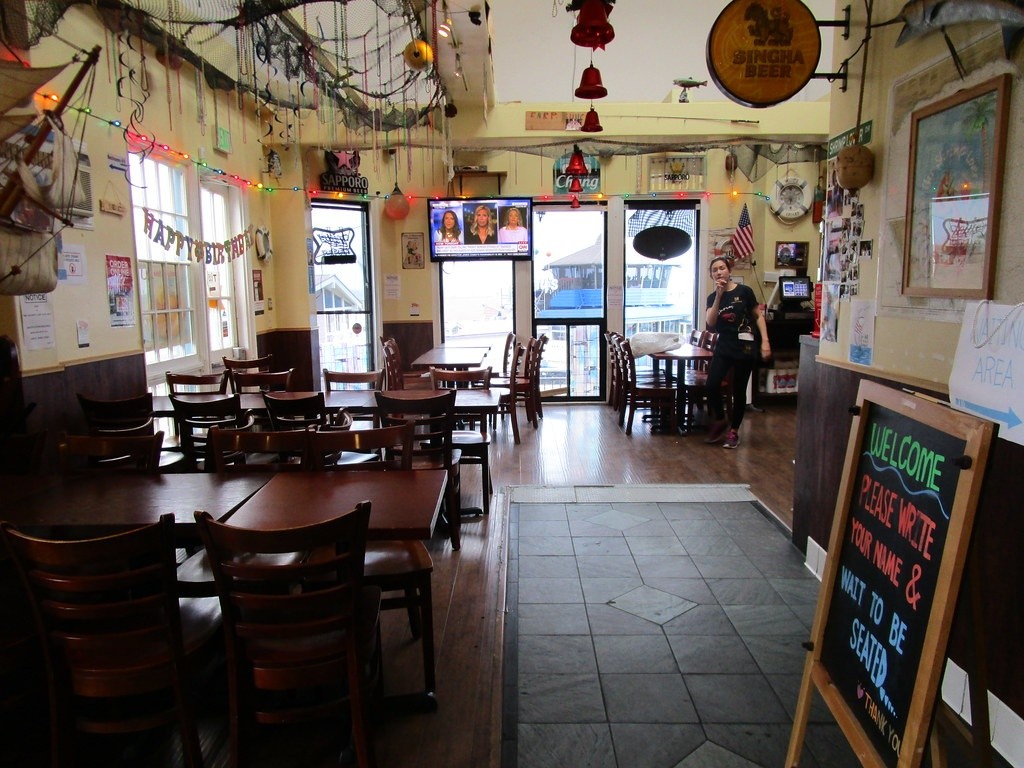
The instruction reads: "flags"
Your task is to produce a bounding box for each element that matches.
[731,204,755,258]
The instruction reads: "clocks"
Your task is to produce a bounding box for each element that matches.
[769,176,812,219]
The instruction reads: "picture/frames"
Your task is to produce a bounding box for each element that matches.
[901,73,1012,300]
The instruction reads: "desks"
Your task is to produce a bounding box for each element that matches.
[639,342,714,361]
[362,389,498,495]
[120,390,376,409]
[225,466,448,639]
[1,466,274,540]
[411,342,491,367]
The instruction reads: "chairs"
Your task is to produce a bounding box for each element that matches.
[0,328,733,768]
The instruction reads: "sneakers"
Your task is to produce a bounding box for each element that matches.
[723,430,740,448]
[705,419,731,442]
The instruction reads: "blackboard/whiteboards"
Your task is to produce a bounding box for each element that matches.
[807,378,1000,768]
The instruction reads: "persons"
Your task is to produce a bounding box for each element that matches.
[465,205,497,244]
[499,208,528,242]
[705,257,770,449]
[827,191,873,298]
[434,210,464,244]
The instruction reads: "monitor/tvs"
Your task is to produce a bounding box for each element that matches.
[427,196,533,262]
[774,241,812,301]
